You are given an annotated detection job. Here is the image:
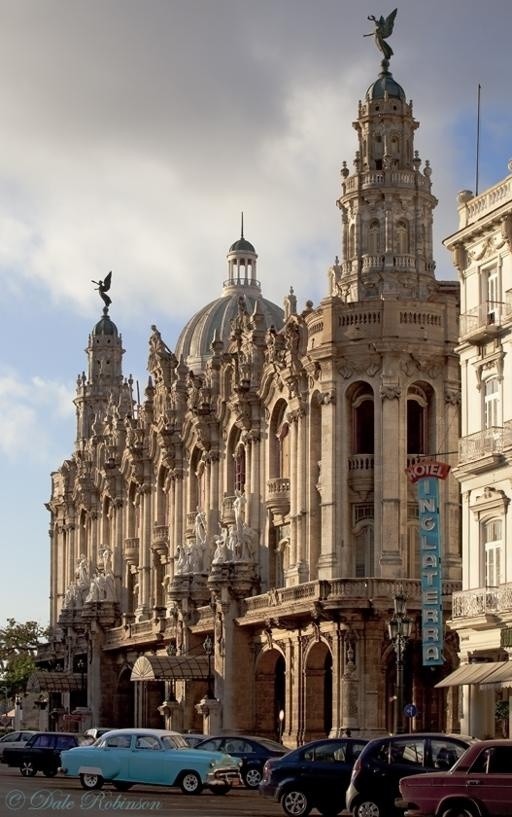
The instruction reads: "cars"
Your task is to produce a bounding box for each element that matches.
[260,737,371,816]
[0,726,290,795]
[346,733,482,817]
[394,737,511,817]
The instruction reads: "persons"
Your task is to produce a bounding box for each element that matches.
[372,15,393,58]
[147,324,165,367]
[61,487,259,608]
[95,279,112,307]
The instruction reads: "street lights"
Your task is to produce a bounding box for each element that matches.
[387,585,414,736]
[203,636,213,699]
[78,660,86,707]
